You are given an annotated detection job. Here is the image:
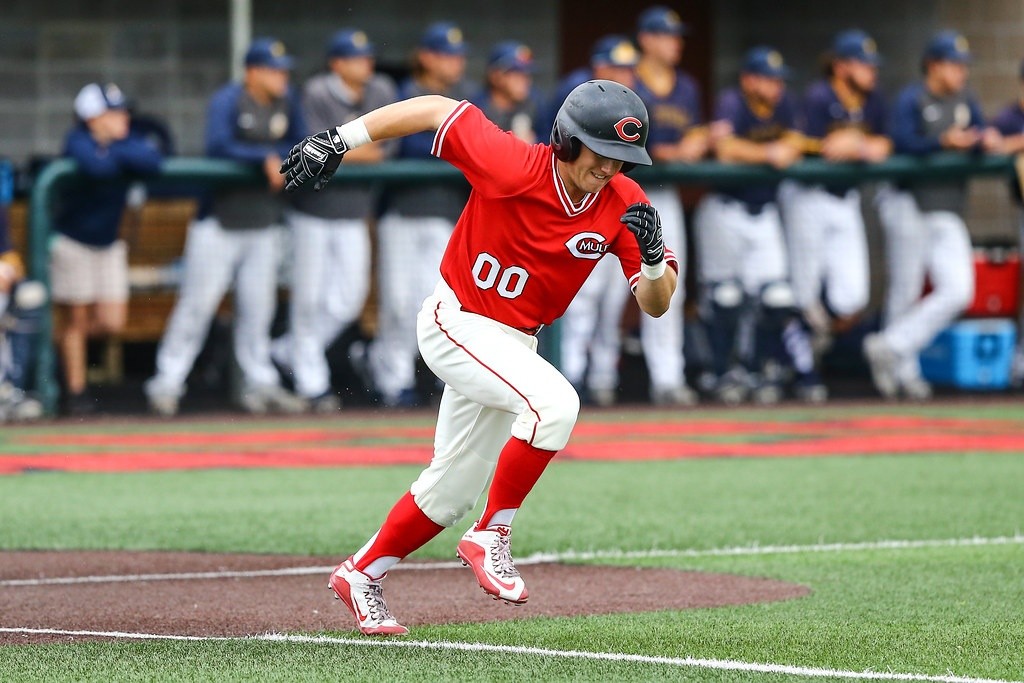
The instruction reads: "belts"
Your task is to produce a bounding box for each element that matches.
[460,305,537,337]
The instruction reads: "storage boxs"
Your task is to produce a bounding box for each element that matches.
[922,244,1020,396]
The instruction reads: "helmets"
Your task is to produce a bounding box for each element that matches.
[549,81,655,173]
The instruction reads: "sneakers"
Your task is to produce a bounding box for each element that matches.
[456,521,529,606]
[328,555,410,637]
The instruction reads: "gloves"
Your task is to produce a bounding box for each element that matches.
[279,117,372,194]
[620,203,665,280]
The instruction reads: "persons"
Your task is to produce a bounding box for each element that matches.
[268,9,1024,413]
[279,78,680,636]
[145,36,310,419]
[0,81,165,421]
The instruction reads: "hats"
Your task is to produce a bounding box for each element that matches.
[420,23,471,56]
[640,9,686,34]
[833,29,886,65]
[72,83,137,120]
[324,27,378,58]
[593,37,640,65]
[244,37,297,68]
[488,39,545,75]
[928,31,977,61]
[740,48,795,79]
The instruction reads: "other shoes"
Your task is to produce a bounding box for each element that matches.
[795,385,830,401]
[717,376,781,405]
[151,394,424,416]
[65,397,102,418]
[0,390,39,417]
[590,391,617,405]
[902,378,932,401]
[863,333,901,400]
[105,392,146,414]
[651,389,698,407]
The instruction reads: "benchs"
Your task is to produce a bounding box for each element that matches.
[10,197,378,378]
